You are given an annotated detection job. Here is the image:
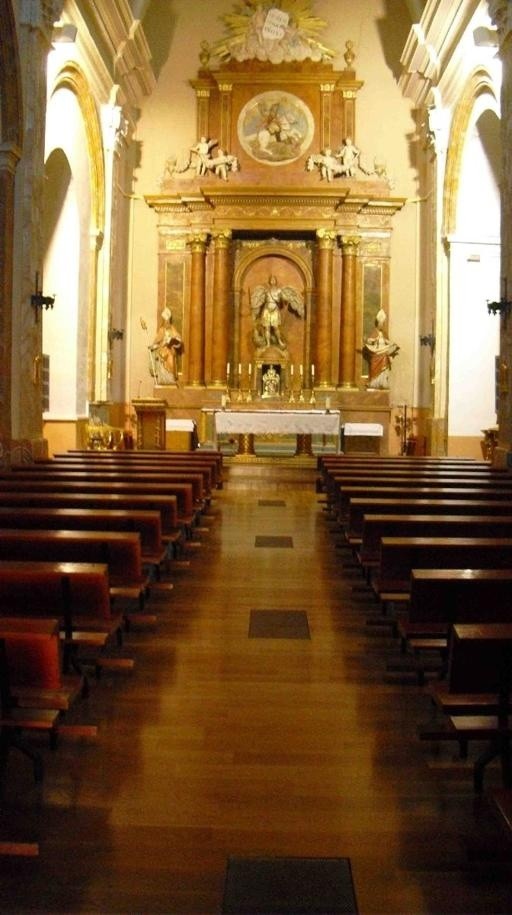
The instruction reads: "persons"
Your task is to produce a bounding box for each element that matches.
[194,137,209,178]
[215,148,227,179]
[337,137,360,174]
[251,275,305,349]
[318,149,336,180]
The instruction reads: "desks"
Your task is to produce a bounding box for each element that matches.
[214,408,341,459]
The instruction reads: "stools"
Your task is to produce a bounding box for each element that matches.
[341,423,383,455]
[165,418,196,453]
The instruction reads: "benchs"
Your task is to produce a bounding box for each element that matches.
[0,446,227,856]
[315,453,511,832]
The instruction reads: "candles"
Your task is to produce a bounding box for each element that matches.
[226,362,316,376]
